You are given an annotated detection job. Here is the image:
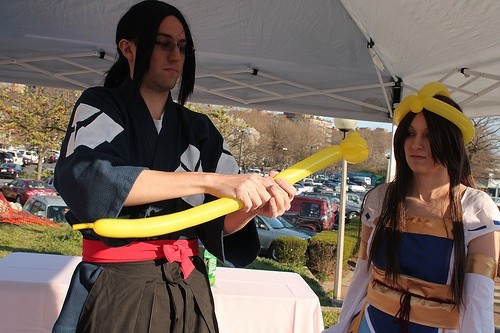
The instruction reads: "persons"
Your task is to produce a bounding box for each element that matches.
[323,93,499,333]
[52,0,298,333]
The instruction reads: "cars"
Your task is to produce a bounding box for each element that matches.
[0,178,59,208]
[0,162,26,179]
[238,166,379,263]
[0,141,71,226]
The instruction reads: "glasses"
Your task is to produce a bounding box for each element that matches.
[154,39,188,54]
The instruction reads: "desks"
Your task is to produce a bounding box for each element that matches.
[0,251,325,333]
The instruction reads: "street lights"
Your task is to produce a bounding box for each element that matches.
[332,117,358,305]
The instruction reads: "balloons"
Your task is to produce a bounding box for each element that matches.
[72,130,369,238]
[393,82,475,144]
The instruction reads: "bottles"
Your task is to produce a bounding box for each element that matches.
[203,249,217,286]
